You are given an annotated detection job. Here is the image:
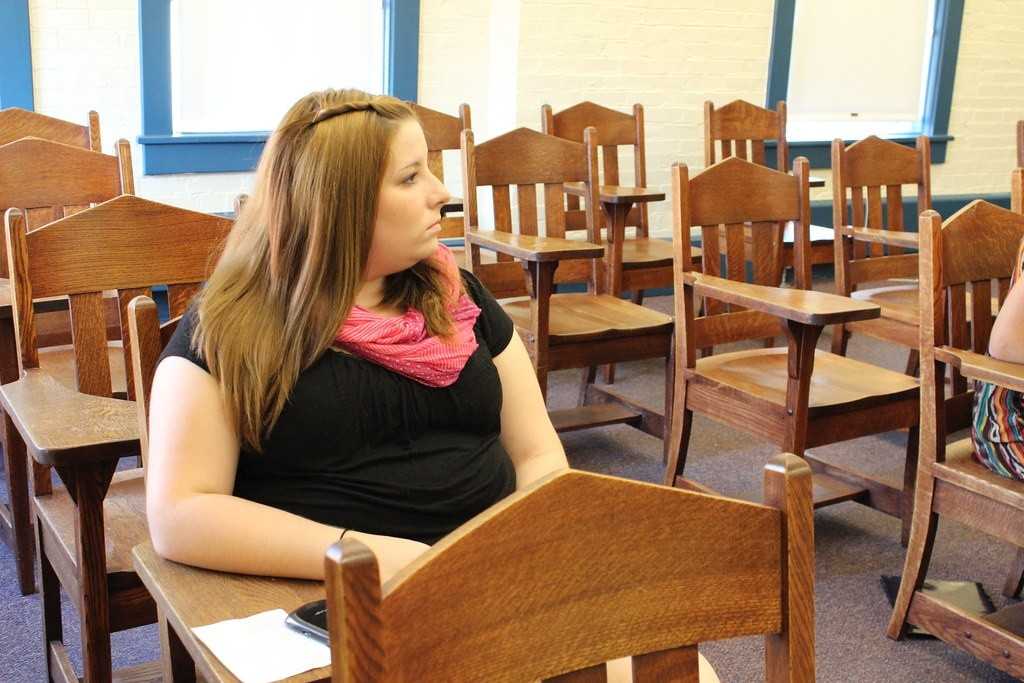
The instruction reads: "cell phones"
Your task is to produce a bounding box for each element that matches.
[284,599,330,648]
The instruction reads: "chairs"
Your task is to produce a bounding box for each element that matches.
[0,107,101,233]
[126,293,333,682]
[0,134,135,594]
[402,98,470,238]
[702,100,854,347]
[0,192,234,682]
[541,101,704,383]
[831,134,1001,378]
[325,450,815,682]
[664,157,920,547]
[460,125,677,464]
[885,197,1024,682]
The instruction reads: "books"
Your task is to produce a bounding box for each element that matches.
[882,574,999,638]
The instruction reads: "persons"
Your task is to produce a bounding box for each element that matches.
[971,236,1024,481]
[146,90,719,683]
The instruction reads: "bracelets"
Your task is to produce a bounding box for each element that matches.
[340,528,349,539]
